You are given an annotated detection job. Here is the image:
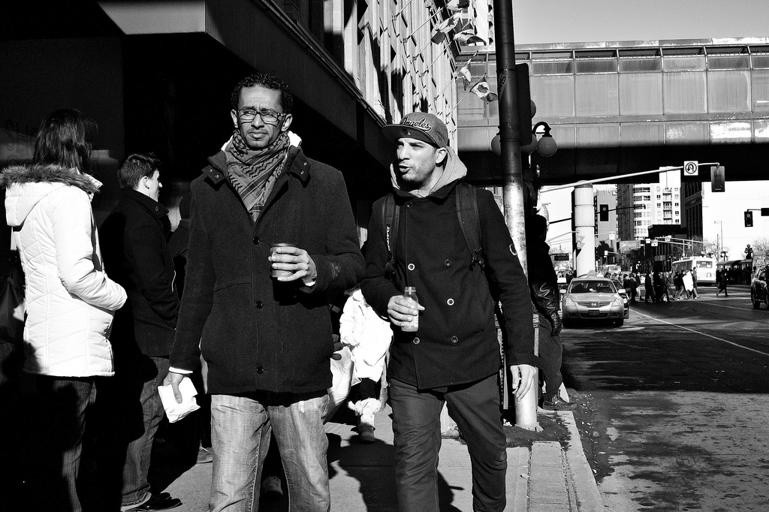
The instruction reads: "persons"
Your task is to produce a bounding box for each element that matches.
[604,266,732,302]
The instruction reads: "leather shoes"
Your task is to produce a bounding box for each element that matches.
[125,492,181,511]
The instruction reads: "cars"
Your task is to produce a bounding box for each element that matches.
[610,276,632,319]
[750,265,769,310]
[559,274,629,330]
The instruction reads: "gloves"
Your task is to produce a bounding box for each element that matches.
[551,312,563,336]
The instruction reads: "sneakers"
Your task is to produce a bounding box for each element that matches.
[542,389,577,411]
[441,423,460,439]
[261,476,283,499]
[359,414,376,442]
[196,439,213,462]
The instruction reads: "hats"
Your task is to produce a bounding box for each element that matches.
[381,113,449,149]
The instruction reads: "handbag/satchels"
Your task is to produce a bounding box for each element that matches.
[0,249,25,338]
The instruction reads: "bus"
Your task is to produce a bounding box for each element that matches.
[670,253,718,286]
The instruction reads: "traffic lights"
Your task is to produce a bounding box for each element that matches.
[744,211,753,228]
[599,203,609,222]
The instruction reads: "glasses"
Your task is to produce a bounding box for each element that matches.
[240,111,286,122]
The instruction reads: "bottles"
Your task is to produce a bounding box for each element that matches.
[401,286,420,332]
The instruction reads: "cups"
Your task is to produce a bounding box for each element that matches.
[269,243,295,279]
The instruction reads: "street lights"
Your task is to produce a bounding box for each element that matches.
[744,244,753,260]
[489,96,559,183]
[714,219,724,249]
[720,251,729,269]
